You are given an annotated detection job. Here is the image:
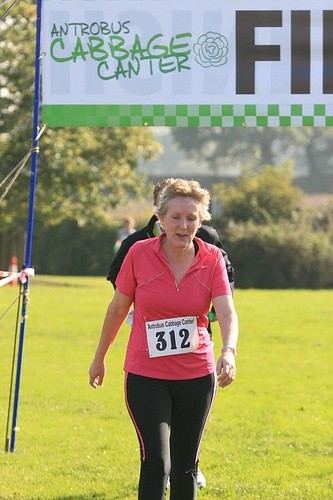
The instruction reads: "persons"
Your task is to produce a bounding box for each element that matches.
[88,178,240,500]
[106,177,237,490]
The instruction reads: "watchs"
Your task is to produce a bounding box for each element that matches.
[222,345,238,357]
